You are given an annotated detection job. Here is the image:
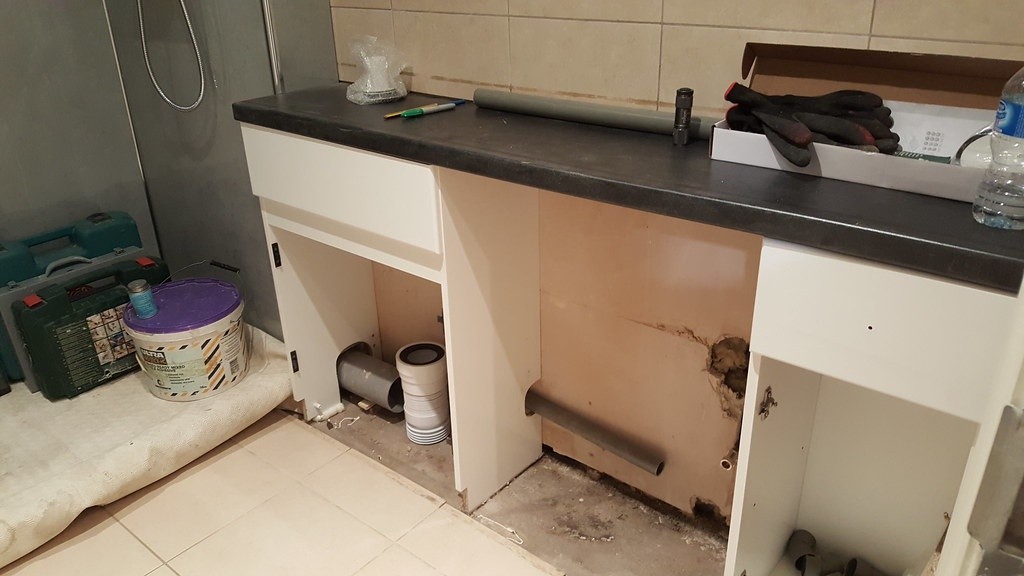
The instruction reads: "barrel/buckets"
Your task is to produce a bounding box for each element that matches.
[122,259,254,401]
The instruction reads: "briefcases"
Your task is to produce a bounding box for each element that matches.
[0,244,143,393]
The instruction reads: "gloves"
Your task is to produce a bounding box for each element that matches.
[725,81,899,167]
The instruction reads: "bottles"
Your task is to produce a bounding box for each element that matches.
[970,62,1024,230]
[126,279,158,319]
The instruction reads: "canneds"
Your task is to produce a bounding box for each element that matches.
[127,279,158,319]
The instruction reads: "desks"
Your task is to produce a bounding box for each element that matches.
[233,82,1024,576]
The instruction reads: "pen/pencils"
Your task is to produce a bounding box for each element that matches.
[400,103,456,119]
[383,100,465,120]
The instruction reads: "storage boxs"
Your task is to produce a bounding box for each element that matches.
[709,42,1024,204]
[0,211,171,403]
